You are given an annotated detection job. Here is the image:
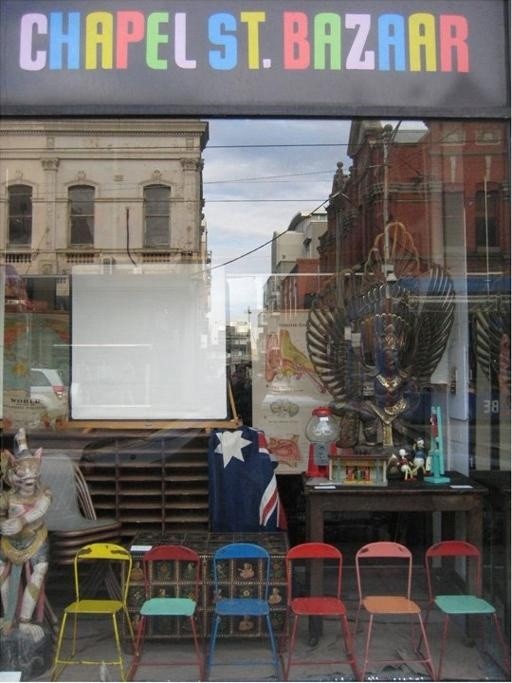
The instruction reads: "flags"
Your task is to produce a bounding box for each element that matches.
[210,425,289,531]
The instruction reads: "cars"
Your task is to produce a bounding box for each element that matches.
[31,367,69,419]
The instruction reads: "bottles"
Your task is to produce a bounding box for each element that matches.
[305,406,341,444]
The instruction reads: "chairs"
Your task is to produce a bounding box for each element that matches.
[278,542,362,682]
[125,545,206,681]
[205,543,285,682]
[34,452,123,654]
[50,543,139,681]
[417,541,511,681]
[351,542,437,681]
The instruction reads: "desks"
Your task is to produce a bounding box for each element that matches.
[300,470,489,648]
